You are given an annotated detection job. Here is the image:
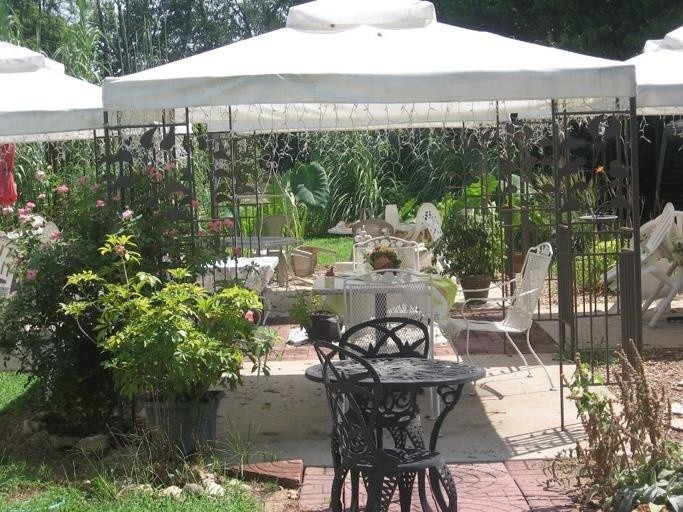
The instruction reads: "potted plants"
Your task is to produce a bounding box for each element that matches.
[48,215,283,470]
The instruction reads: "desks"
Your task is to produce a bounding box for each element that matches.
[304,357,486,511]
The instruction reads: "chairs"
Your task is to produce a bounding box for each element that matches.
[312,338,458,511]
[338,318,431,512]
